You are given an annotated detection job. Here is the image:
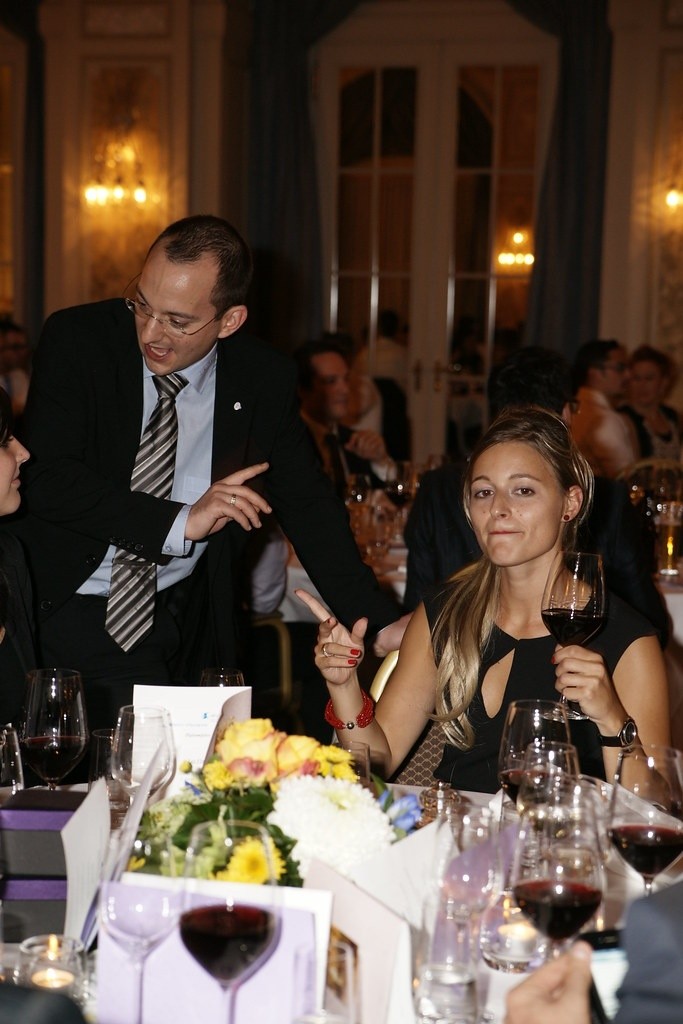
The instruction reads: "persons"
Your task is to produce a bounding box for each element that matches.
[0,311,683,1024]
[22,215,414,785]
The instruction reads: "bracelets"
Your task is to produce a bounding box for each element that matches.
[323,686,375,729]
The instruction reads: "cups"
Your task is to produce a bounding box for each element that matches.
[332,742,371,790]
[0,668,176,828]
[659,519,681,572]
[199,666,244,686]
[409,799,553,1024]
[14,933,88,1011]
[290,939,356,1024]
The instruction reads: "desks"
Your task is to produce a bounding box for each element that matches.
[287,543,406,626]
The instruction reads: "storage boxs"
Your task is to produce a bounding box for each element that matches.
[0,790,87,942]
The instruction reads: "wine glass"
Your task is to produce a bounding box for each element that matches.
[178,819,284,1024]
[540,551,605,721]
[497,699,683,965]
[97,827,180,1023]
[342,454,449,573]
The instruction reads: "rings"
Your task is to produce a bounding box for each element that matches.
[321,643,334,657]
[230,494,235,504]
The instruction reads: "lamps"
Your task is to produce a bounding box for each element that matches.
[83,76,152,203]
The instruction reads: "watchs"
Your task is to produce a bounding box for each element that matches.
[601,716,639,749]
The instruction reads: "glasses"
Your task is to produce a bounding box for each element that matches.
[122,272,237,335]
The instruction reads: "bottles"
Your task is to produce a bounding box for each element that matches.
[413,781,468,827]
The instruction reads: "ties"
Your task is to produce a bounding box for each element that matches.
[103,372,190,655]
[322,432,348,500]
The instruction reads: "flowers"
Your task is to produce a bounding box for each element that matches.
[122,709,404,894]
